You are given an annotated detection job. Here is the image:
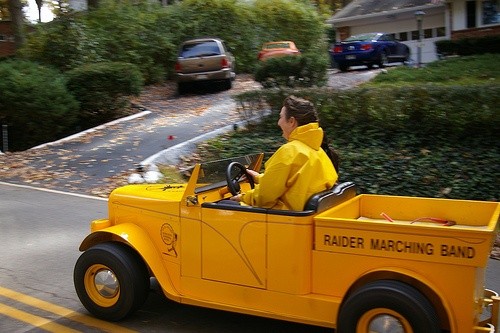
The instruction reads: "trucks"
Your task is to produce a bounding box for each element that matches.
[74,151,500,332]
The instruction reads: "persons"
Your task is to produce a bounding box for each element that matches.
[230,96,338,212]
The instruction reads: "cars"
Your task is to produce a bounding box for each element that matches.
[329,32,410,71]
[176,37,236,92]
[258,41,304,62]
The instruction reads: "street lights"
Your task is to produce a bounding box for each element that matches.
[413,11,426,67]
